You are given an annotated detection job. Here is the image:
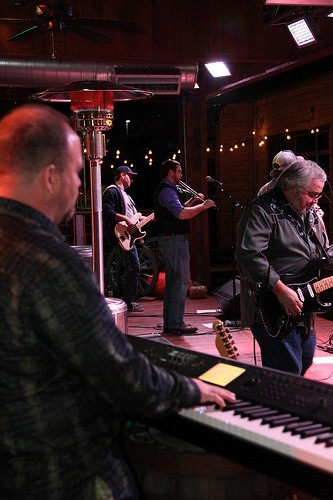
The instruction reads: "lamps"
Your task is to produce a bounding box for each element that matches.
[286,6,322,48]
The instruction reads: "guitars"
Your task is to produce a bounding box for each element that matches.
[246,258,332,344]
[113,211,155,252]
[212,319,239,360]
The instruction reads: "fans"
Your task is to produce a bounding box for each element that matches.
[0,0,143,59]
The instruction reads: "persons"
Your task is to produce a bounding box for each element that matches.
[153,161,216,334]
[235,150,333,380]
[103,166,157,312]
[0,103,236,500]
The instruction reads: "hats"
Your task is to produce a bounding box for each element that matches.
[114,166,137,176]
[272,150,305,170]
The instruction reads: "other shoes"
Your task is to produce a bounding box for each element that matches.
[163,324,198,334]
[127,301,144,312]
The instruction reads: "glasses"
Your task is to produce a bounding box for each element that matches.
[301,186,323,199]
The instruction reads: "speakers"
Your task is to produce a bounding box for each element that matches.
[207,275,242,319]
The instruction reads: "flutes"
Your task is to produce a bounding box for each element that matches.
[179,181,220,210]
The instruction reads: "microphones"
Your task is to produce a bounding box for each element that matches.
[205,176,223,185]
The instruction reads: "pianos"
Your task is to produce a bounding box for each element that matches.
[121,331,333,500]
